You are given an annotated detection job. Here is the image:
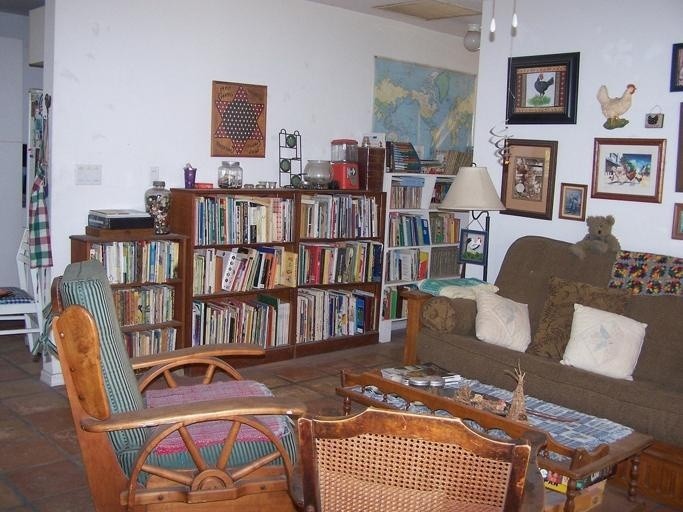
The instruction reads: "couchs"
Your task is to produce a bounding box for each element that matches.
[394,232,683,511]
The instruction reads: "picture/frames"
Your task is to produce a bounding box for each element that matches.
[498,138,559,221]
[590,135,668,205]
[558,182,588,222]
[670,42,683,92]
[455,227,489,268]
[504,51,581,125]
[671,202,683,241]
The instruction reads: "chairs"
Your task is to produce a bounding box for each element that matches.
[47,258,312,511]
[292,402,533,511]
[0,227,46,356]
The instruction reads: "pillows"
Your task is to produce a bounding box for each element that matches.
[526,273,635,361]
[418,293,477,336]
[473,283,533,355]
[558,301,651,383]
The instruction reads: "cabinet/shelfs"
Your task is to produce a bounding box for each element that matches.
[376,172,470,345]
[166,184,389,380]
[65,228,189,380]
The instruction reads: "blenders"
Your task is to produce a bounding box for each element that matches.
[330,139,360,190]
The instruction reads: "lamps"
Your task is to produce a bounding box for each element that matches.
[462,22,482,52]
[434,162,508,282]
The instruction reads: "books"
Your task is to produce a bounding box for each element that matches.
[381,181,461,320]
[192,195,298,348]
[295,194,384,345]
[89,239,180,360]
[387,143,472,175]
[381,363,462,386]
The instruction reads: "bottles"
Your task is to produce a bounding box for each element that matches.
[408,376,447,405]
[216,160,244,188]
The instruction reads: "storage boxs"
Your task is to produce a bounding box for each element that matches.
[343,145,385,192]
[538,462,618,497]
[545,478,608,512]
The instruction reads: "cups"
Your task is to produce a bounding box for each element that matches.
[183,167,196,189]
[258,180,277,189]
[302,159,335,190]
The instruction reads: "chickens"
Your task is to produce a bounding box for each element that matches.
[595,83,637,127]
[466,237,481,253]
